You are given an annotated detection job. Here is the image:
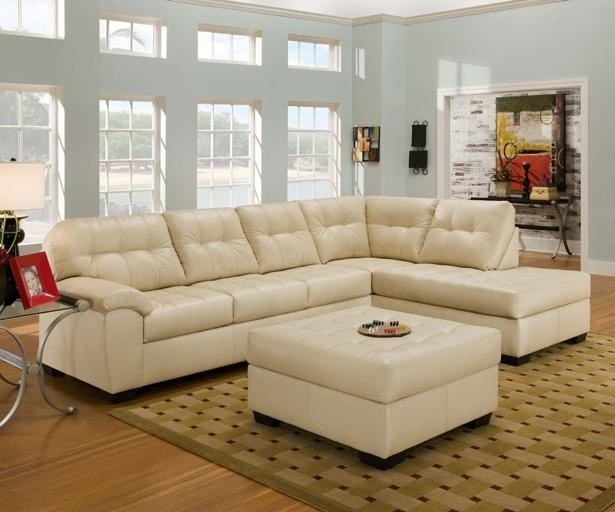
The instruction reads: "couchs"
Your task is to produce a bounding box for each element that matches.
[38,196,591,404]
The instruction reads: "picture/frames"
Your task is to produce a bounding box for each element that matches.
[9,251,61,309]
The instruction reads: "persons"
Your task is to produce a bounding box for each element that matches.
[22,267,42,296]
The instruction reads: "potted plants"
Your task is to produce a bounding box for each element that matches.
[492,149,514,195]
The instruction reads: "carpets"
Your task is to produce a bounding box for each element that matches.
[107,331,614,512]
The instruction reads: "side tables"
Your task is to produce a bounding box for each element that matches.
[1,295,88,428]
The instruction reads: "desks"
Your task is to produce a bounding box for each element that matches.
[470,195,573,258]
